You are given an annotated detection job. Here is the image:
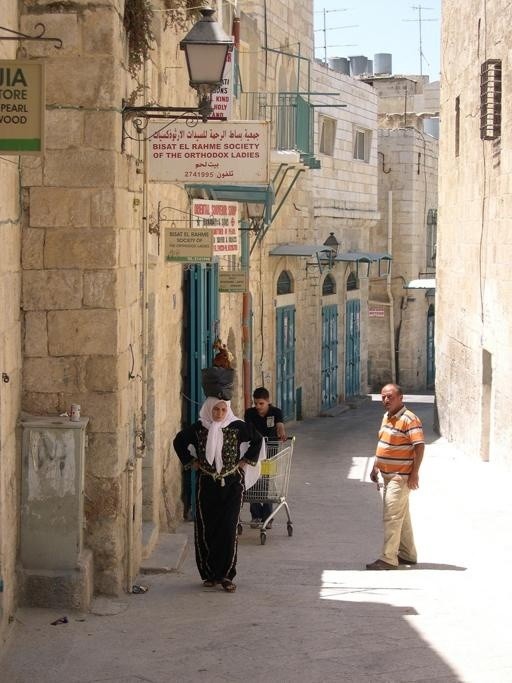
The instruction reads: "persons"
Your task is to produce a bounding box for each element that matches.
[363,381,425,571]
[242,386,288,531]
[171,395,265,593]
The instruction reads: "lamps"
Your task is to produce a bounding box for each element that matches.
[305,231,339,276]
[121,4,234,153]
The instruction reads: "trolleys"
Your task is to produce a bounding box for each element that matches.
[236,437,296,546]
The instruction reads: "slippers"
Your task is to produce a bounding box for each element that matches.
[204,578,223,587]
[221,580,236,592]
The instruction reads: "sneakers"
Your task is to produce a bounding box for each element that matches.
[364,558,397,569]
[396,553,417,565]
[250,517,273,529]
